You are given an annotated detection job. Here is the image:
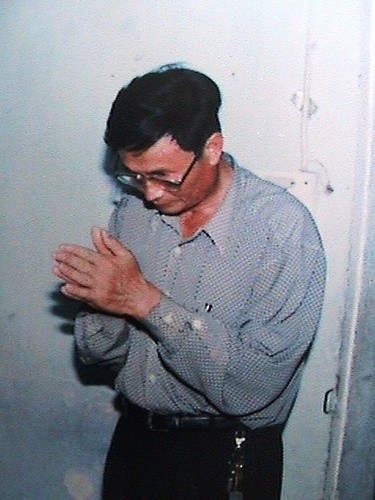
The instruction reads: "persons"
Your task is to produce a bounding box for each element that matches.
[50,64,327,500]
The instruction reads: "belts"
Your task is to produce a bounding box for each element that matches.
[120,399,237,435]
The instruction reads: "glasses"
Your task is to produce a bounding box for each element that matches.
[109,150,203,192]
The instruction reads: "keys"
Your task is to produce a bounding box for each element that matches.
[226,429,246,498]
